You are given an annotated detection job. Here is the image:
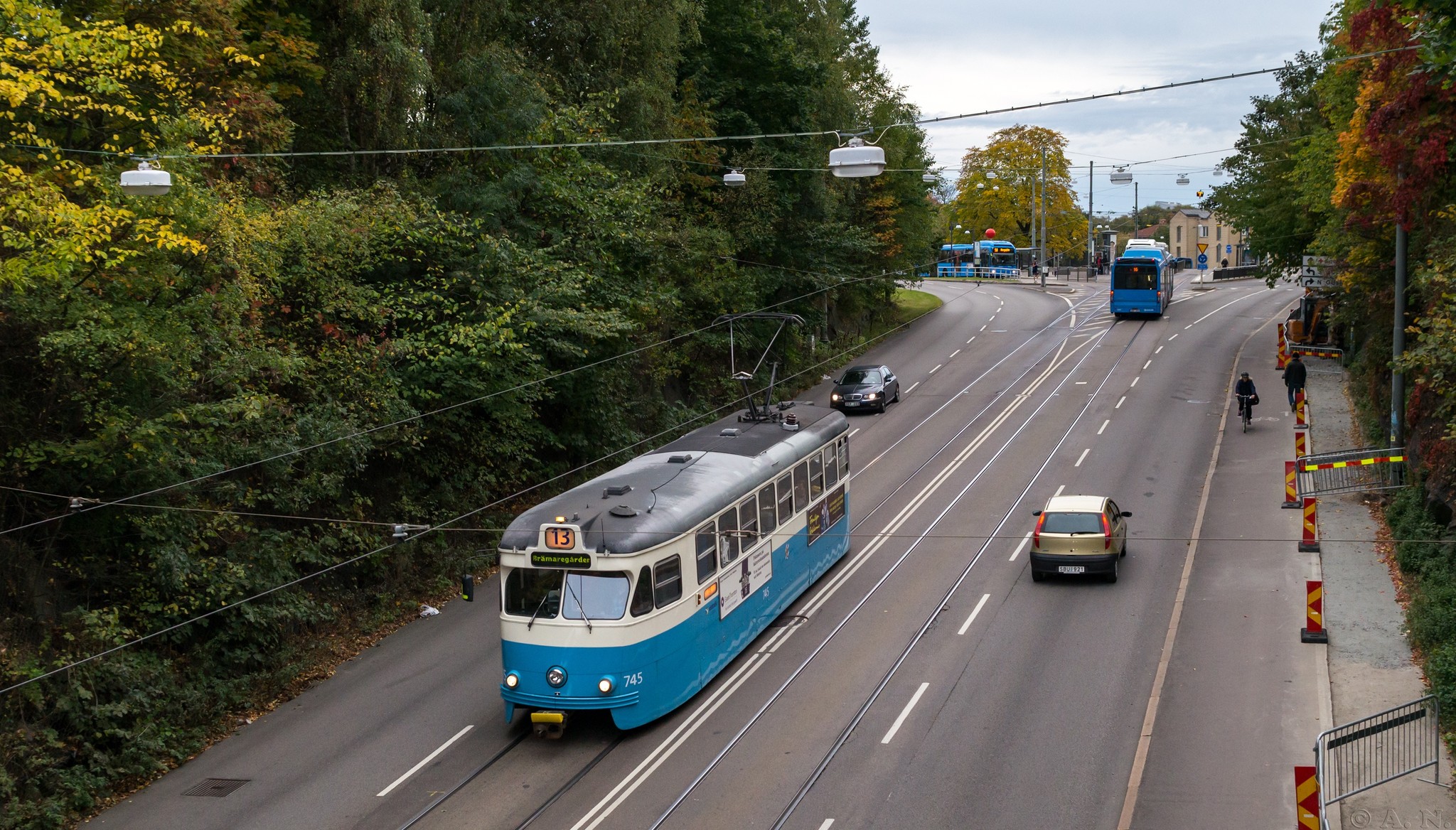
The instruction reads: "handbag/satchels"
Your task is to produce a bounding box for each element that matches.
[1250,392,1259,405]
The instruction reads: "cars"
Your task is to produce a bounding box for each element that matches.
[829,364,902,414]
[1030,495,1135,583]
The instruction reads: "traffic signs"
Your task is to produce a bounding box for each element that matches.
[1301,277,1341,288]
[1302,266,1340,277]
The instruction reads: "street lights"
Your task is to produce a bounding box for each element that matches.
[965,229,975,242]
[950,222,963,277]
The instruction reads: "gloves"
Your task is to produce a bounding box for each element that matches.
[1285,380,1288,386]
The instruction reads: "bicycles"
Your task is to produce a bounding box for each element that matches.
[1235,394,1257,433]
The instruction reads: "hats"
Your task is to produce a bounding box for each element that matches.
[1292,352,1300,358]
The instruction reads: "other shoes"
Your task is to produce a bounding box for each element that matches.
[1291,402,1296,412]
[1238,410,1242,416]
[1247,420,1251,425]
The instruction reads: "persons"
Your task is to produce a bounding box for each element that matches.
[1032,261,1039,284]
[1096,255,1108,275]
[1284,352,1307,412]
[1236,371,1255,425]
[795,479,808,509]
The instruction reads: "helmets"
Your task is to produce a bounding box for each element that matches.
[1241,372,1249,378]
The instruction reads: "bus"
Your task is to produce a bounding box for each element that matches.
[1109,238,1177,321]
[940,240,1018,277]
[881,253,931,277]
[461,310,853,741]
[1016,248,1042,272]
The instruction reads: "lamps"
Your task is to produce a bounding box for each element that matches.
[1176,174,1189,185]
[1214,170,1223,176]
[986,171,997,179]
[977,183,984,188]
[828,135,886,178]
[1227,174,1234,178]
[1109,167,1133,184]
[922,169,935,182]
[724,169,747,188]
[993,186,999,190]
[118,156,174,196]
